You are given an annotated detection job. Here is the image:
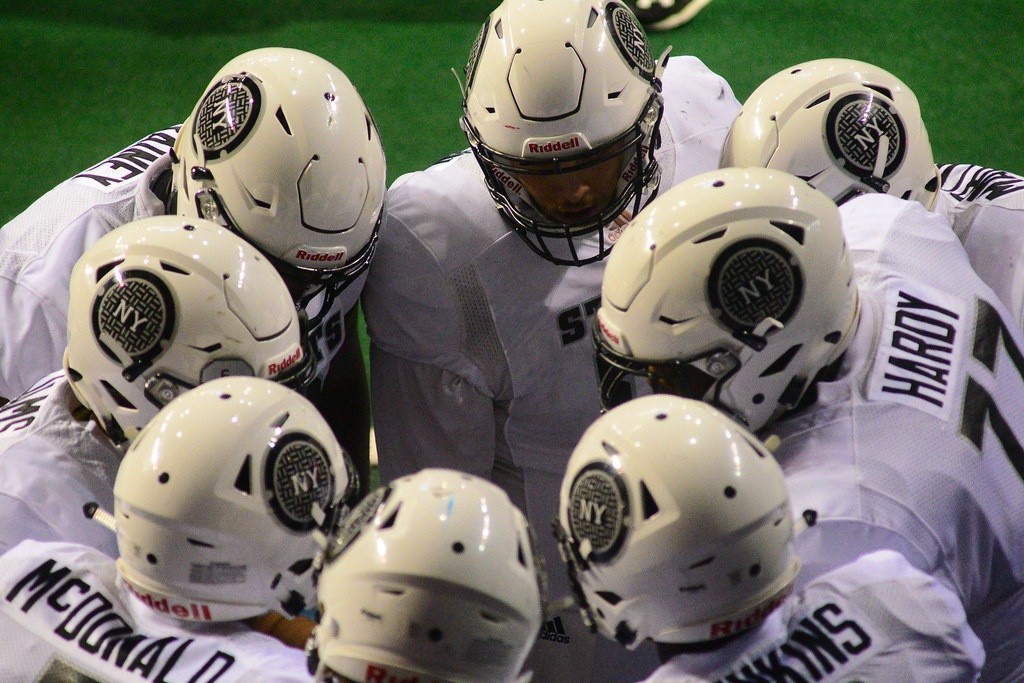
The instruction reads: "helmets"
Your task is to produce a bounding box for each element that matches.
[719,58,941,212]
[304,468,542,683]
[92,375,350,624]
[552,394,803,652]
[168,48,388,329]
[463,0,672,266]
[597,167,862,431]
[63,214,303,452]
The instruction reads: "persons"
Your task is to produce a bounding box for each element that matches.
[0,47,387,683]
[552,59,1024,683]
[305,466,549,683]
[361,0,743,683]
